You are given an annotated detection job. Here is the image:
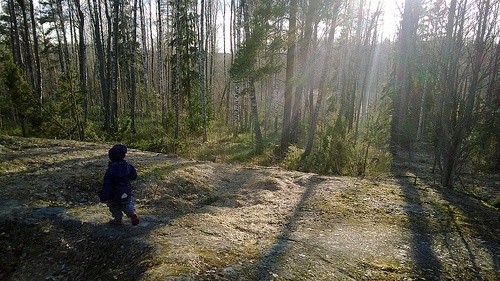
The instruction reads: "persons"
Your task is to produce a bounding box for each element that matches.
[101,144,139,227]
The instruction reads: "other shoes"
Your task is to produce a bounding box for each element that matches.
[109,218,122,225]
[131,214,139,225]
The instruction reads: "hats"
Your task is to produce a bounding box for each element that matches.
[108,145,127,161]
